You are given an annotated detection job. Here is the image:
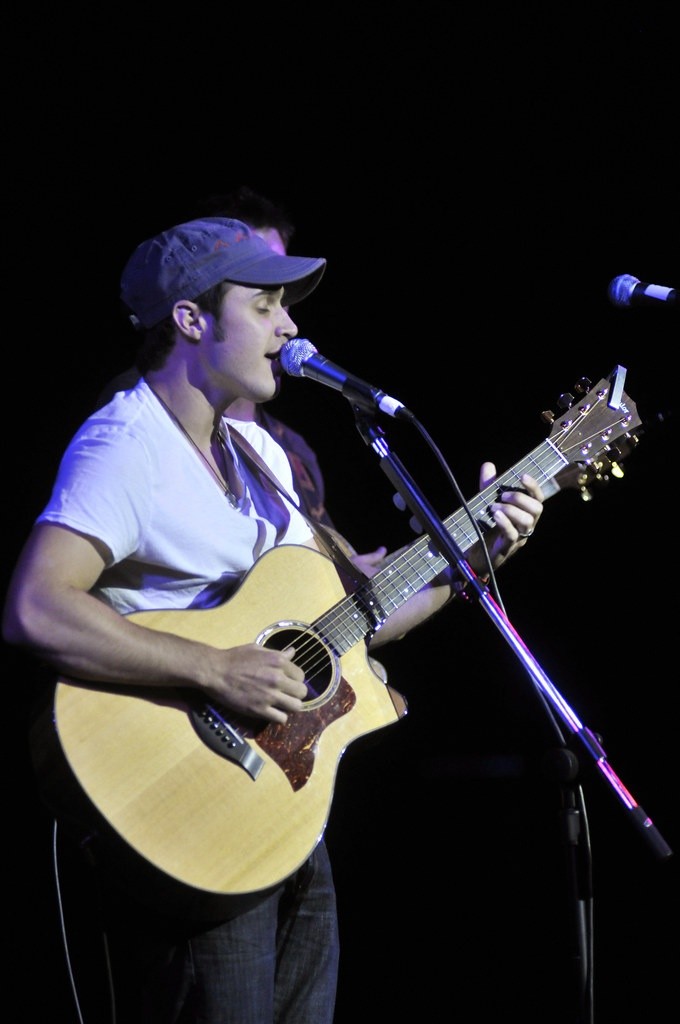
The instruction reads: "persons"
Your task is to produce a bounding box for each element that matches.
[14,214,544,1024]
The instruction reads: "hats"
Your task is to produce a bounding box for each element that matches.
[118,216,329,331]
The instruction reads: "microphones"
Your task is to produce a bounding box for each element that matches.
[608,274,680,306]
[281,338,414,421]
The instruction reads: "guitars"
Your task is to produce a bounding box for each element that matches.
[49,367,646,897]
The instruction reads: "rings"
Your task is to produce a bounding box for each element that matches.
[519,526,534,538]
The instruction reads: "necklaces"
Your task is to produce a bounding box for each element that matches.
[145,380,239,509]
